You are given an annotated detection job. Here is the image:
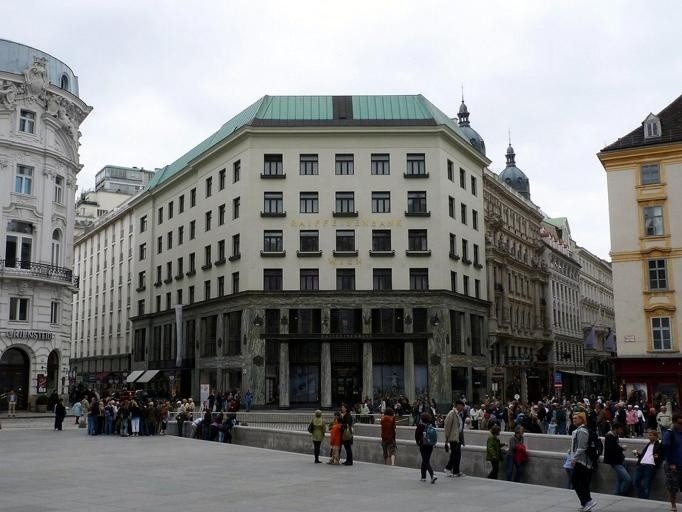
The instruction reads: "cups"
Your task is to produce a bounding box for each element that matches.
[623,445,628,451]
[632,449,638,458]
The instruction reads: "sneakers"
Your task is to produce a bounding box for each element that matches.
[430,468,462,484]
[578,500,597,512]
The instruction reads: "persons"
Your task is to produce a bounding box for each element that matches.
[632,430,662,499]
[328,403,353,466]
[412,395,437,427]
[506,425,528,483]
[6,390,17,417]
[415,413,437,483]
[381,408,396,465]
[309,410,326,464]
[485,425,508,479]
[354,392,411,423]
[562,412,598,511]
[661,413,682,512]
[603,423,632,496]
[50,389,253,444]
[459,392,674,439]
[443,400,465,477]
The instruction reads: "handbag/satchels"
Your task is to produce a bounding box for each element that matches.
[308,423,314,433]
[342,429,354,440]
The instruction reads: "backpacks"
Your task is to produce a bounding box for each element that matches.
[420,423,437,446]
[573,426,602,461]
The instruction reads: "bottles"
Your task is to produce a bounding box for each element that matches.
[423,432,426,438]
[590,441,596,448]
